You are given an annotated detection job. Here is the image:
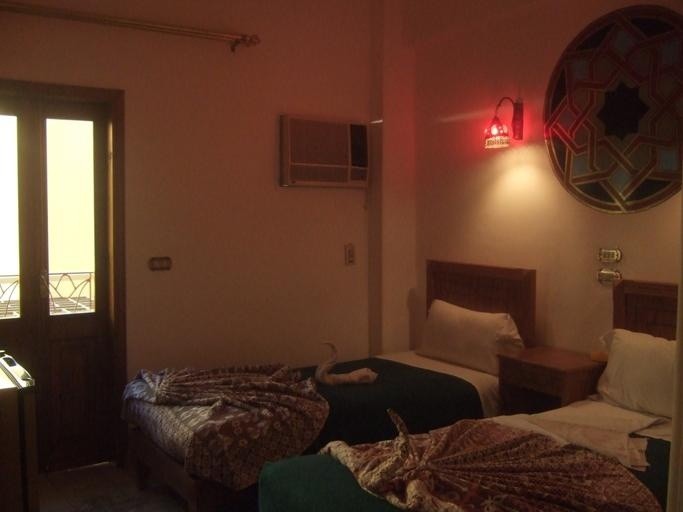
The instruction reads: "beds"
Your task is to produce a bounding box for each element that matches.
[242,276,680,508]
[125,262,540,508]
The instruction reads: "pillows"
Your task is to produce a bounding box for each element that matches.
[591,324,680,422]
[413,296,517,377]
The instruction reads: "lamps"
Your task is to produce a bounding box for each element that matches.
[482,95,525,149]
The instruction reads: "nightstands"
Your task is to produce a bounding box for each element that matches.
[493,344,600,413]
